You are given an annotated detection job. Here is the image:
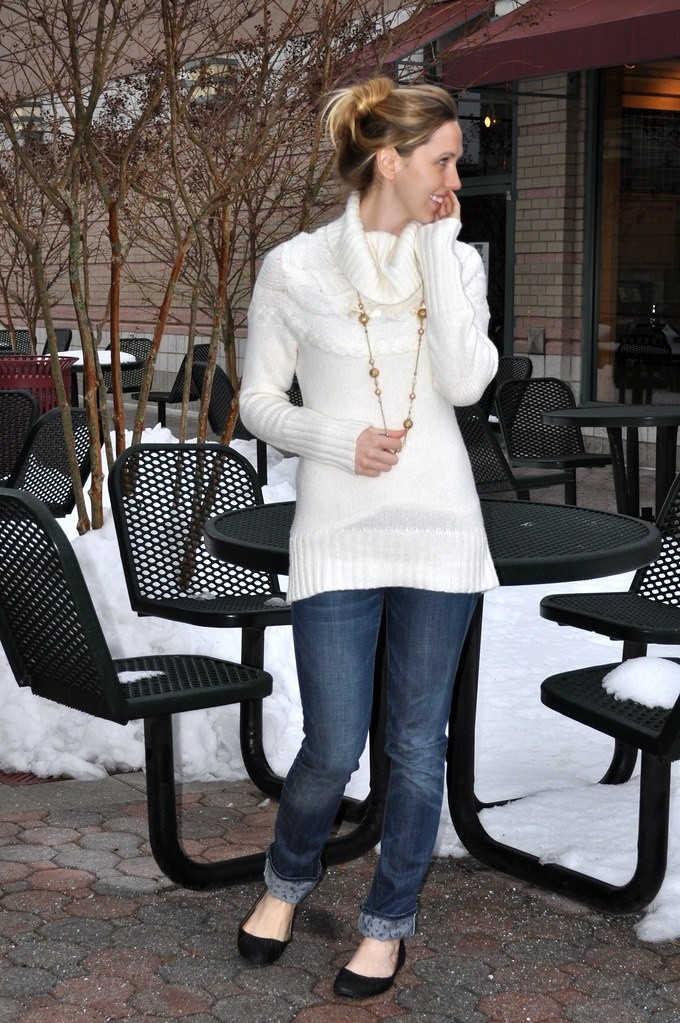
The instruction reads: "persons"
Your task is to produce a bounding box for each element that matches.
[239,72,500,1000]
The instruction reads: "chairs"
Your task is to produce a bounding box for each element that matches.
[611,324,672,402]
[453,403,573,493]
[192,361,257,442]
[540,470,680,643]
[131,342,211,403]
[0,329,37,356]
[41,327,74,357]
[98,337,153,394]
[0,407,109,518]
[491,357,534,433]
[0,483,273,726]
[494,378,613,468]
[107,441,297,628]
[0,388,37,489]
[540,655,680,761]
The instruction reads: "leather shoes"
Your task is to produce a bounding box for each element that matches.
[318,917,406,1000]
[238,887,298,964]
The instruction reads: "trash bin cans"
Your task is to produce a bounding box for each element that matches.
[0,352,79,419]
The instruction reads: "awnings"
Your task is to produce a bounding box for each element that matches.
[433,0,679,85]
[316,0,494,72]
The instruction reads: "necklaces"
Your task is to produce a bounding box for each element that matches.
[356,291,430,452]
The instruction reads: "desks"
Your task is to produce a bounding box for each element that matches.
[541,406,680,512]
[142,500,672,913]
[71,354,143,408]
